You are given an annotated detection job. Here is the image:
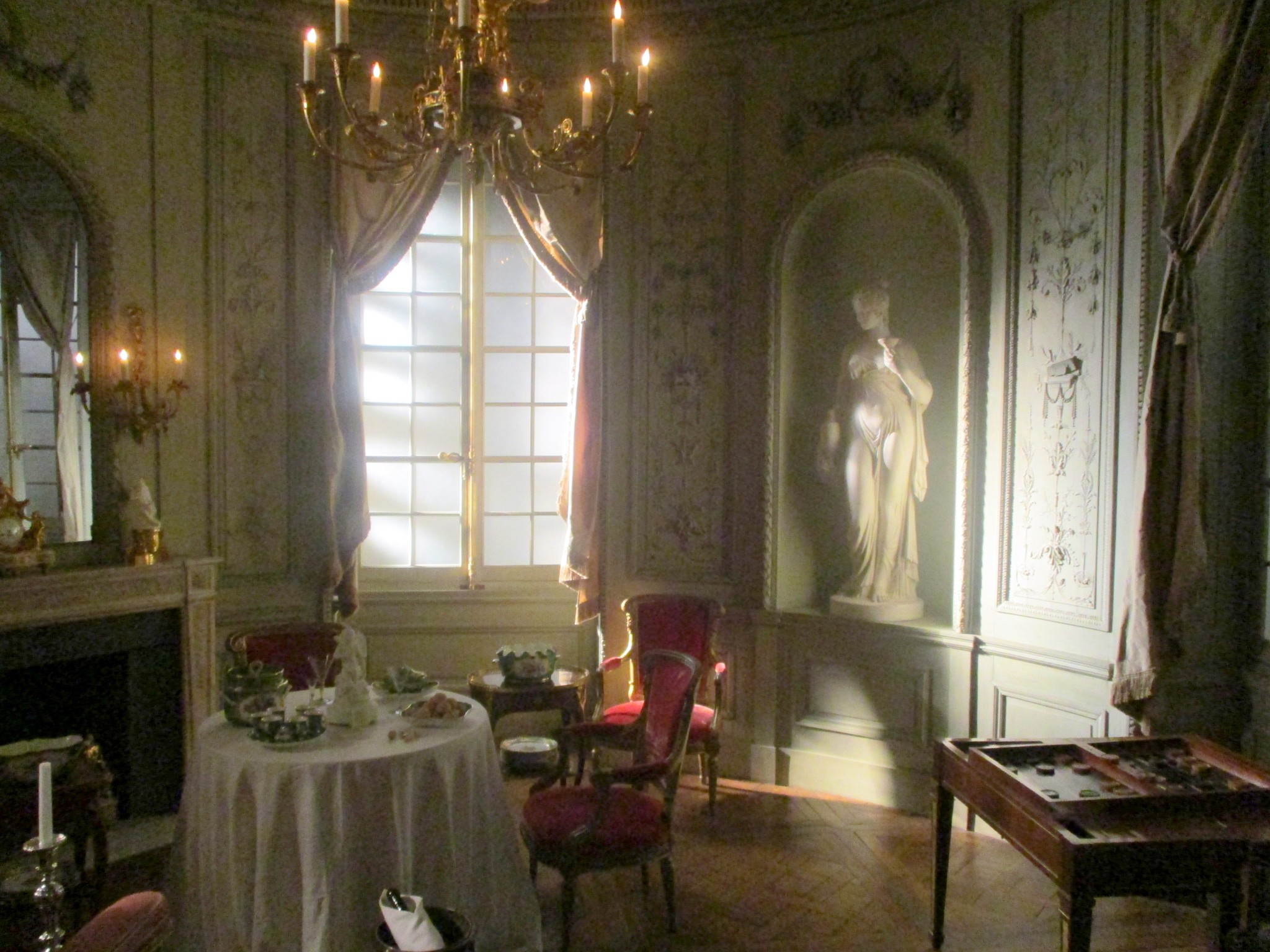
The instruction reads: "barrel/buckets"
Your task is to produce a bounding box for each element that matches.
[376,907,474,952]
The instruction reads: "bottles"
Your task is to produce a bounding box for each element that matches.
[387,888,409,911]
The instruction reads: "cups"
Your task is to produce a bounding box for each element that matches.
[290,704,323,739]
[250,707,285,742]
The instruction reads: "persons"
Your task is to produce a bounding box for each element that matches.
[333,627,366,685]
[819,286,933,603]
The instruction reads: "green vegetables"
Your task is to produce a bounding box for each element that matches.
[376,666,438,692]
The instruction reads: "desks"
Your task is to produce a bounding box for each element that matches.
[931,732,1270,952]
[172,686,546,952]
[469,660,588,786]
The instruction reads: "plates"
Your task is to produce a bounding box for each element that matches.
[371,682,439,701]
[247,721,327,746]
[402,701,473,724]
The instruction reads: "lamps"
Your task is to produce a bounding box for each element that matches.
[288,0,669,194]
[71,307,189,443]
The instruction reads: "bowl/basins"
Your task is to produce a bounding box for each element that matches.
[492,643,560,683]
[223,664,288,725]
[500,736,559,773]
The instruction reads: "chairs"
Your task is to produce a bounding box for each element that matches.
[518,650,707,949]
[574,591,725,818]
[227,621,368,693]
[67,892,169,952]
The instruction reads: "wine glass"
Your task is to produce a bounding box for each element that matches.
[307,653,336,708]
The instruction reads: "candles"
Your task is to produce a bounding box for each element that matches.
[36,762,56,847]
